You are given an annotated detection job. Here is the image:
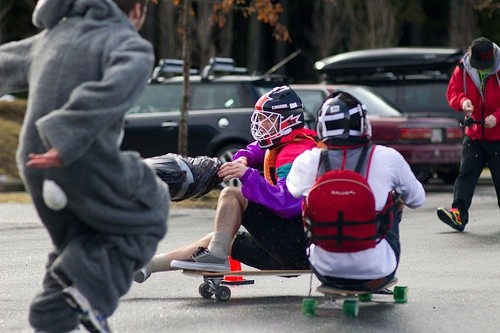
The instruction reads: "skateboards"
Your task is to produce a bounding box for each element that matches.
[180,267,315,302]
[300,277,409,317]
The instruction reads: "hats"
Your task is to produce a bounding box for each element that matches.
[469,37,494,70]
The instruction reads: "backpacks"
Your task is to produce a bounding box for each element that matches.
[301,142,394,253]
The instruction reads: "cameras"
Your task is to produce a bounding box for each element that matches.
[464,116,483,127]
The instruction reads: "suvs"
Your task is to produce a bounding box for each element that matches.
[120,57,289,190]
[313,48,500,184]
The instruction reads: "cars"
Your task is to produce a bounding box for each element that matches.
[291,85,464,184]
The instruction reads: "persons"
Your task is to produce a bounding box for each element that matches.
[285,91,426,292]
[0,1,170,333]
[132,85,327,283]
[436,36,500,233]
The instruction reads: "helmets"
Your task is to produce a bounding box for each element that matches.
[317,91,372,145]
[250,85,304,149]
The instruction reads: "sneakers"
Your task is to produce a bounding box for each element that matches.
[133,266,151,283]
[61,284,112,333]
[437,207,465,231]
[170,247,230,273]
